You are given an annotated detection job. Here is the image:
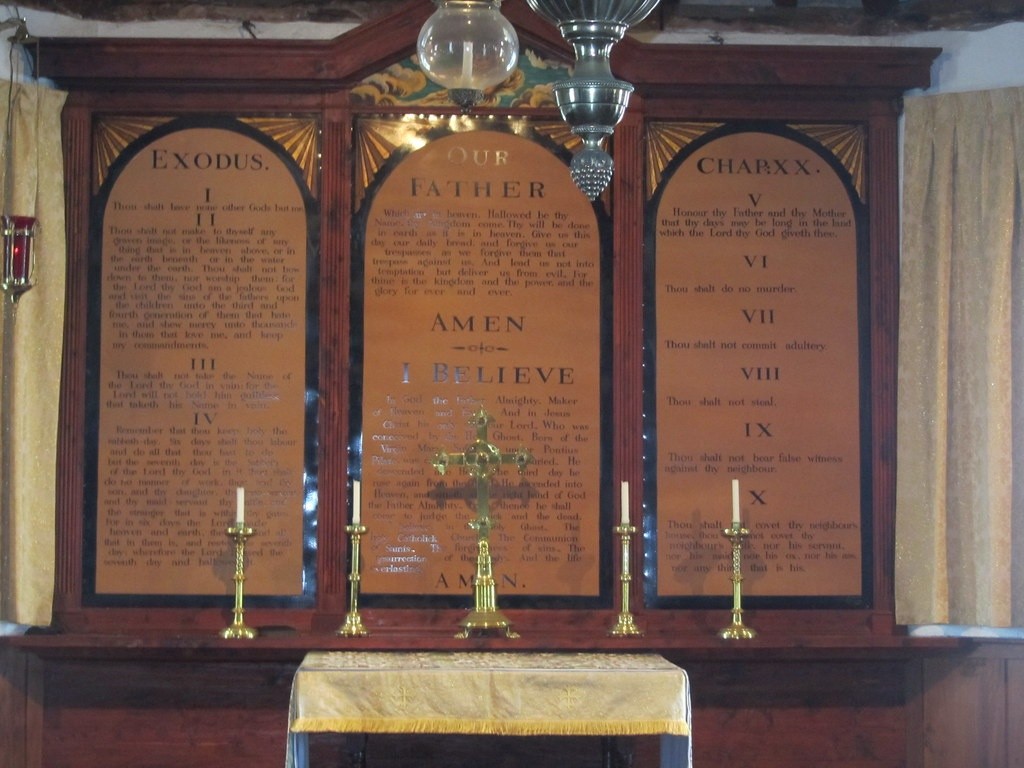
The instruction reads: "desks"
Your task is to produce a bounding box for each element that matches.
[285,651,692,768]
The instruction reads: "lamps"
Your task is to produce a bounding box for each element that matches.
[0,18,35,303]
[416,0,662,201]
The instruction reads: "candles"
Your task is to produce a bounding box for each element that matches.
[352,480,360,523]
[237,487,244,522]
[621,481,629,523]
[733,479,739,522]
